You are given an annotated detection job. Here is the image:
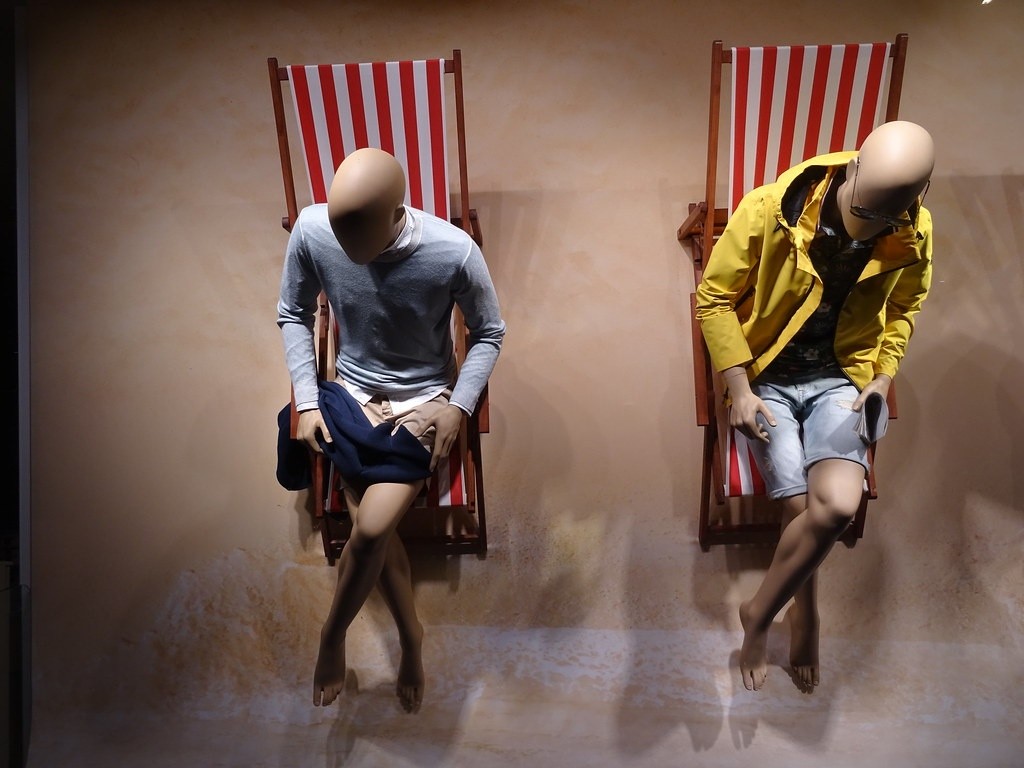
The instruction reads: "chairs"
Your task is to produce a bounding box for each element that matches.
[266,49,492,563]
[674,33,908,553]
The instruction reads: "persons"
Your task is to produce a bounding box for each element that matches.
[277,148,507,715]
[694,119,935,693]
